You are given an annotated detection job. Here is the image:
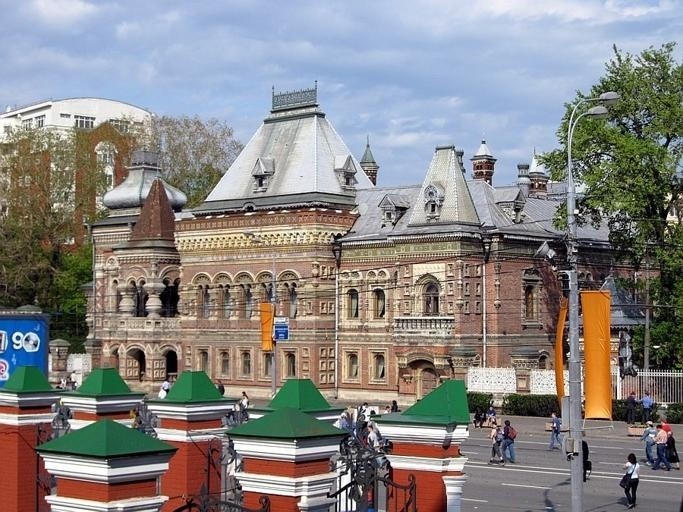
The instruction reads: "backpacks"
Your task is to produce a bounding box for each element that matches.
[495,428,504,442]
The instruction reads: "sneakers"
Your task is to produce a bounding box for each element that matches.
[628,503,634,509]
[646,461,680,471]
[488,458,505,466]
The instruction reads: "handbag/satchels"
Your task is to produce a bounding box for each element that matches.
[619,473,632,488]
[585,461,591,470]
[508,426,518,439]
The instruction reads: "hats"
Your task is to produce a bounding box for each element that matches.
[646,421,653,425]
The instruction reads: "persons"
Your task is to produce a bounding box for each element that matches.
[359,400,397,450]
[502,420,514,463]
[55,370,249,429]
[623,453,639,509]
[548,412,562,450]
[640,418,680,471]
[487,406,497,425]
[473,406,486,428]
[639,391,652,424]
[582,431,589,482]
[626,392,636,423]
[488,422,504,465]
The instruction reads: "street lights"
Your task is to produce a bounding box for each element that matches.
[567,92,620,512]
[330,232,343,400]
[81,233,95,338]
[242,230,276,393]
[481,235,491,368]
